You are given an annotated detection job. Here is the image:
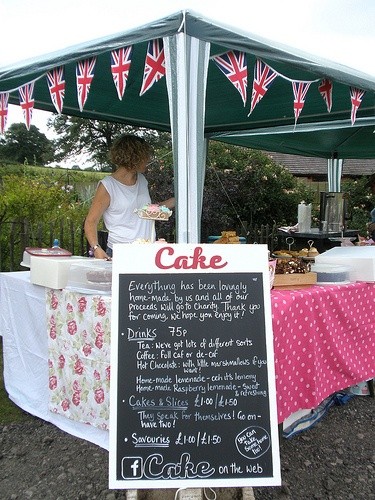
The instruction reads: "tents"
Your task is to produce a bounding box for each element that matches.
[0,11,375,252]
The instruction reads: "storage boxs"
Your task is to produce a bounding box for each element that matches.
[66,258,113,295]
[314,245,375,281]
[310,263,352,281]
[30,255,71,290]
[273,271,317,290]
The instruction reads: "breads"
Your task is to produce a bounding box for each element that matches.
[215,231,241,244]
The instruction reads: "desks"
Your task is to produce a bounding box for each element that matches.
[271,281,375,425]
[0,271,111,452]
[277,230,361,250]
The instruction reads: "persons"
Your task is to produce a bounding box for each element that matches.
[84,134,175,258]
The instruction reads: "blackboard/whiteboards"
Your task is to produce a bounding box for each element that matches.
[107,267,283,490]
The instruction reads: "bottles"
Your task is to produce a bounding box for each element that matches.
[52,239,61,249]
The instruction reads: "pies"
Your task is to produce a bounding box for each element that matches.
[274,250,299,258]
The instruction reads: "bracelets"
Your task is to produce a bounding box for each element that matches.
[89,244,100,255]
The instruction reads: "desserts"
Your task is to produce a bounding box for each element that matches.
[298,247,318,258]
[138,204,172,219]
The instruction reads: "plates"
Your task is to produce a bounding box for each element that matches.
[137,214,169,221]
